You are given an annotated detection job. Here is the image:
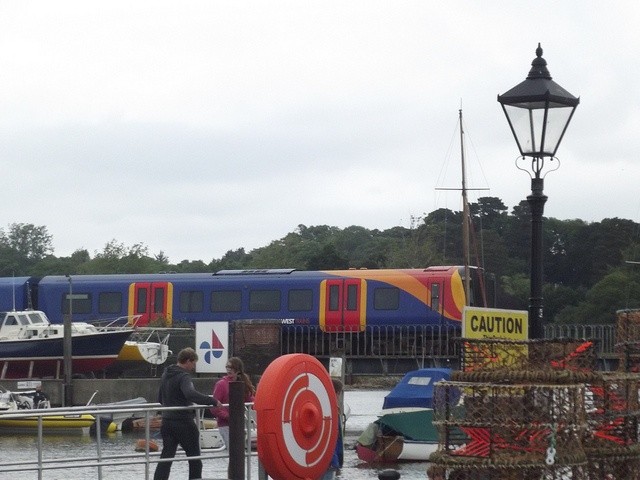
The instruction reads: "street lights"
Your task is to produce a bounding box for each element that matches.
[496,44,579,365]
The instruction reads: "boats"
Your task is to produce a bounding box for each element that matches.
[0,384,96,436]
[83,396,147,431]
[174,409,259,452]
[354,404,596,467]
[0,311,142,381]
[114,330,173,364]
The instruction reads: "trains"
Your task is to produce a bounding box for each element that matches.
[0,266,496,351]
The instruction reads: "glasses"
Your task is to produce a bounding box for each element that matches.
[225,365,232,368]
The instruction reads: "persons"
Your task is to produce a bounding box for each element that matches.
[210,356,257,453]
[153,346,223,480]
